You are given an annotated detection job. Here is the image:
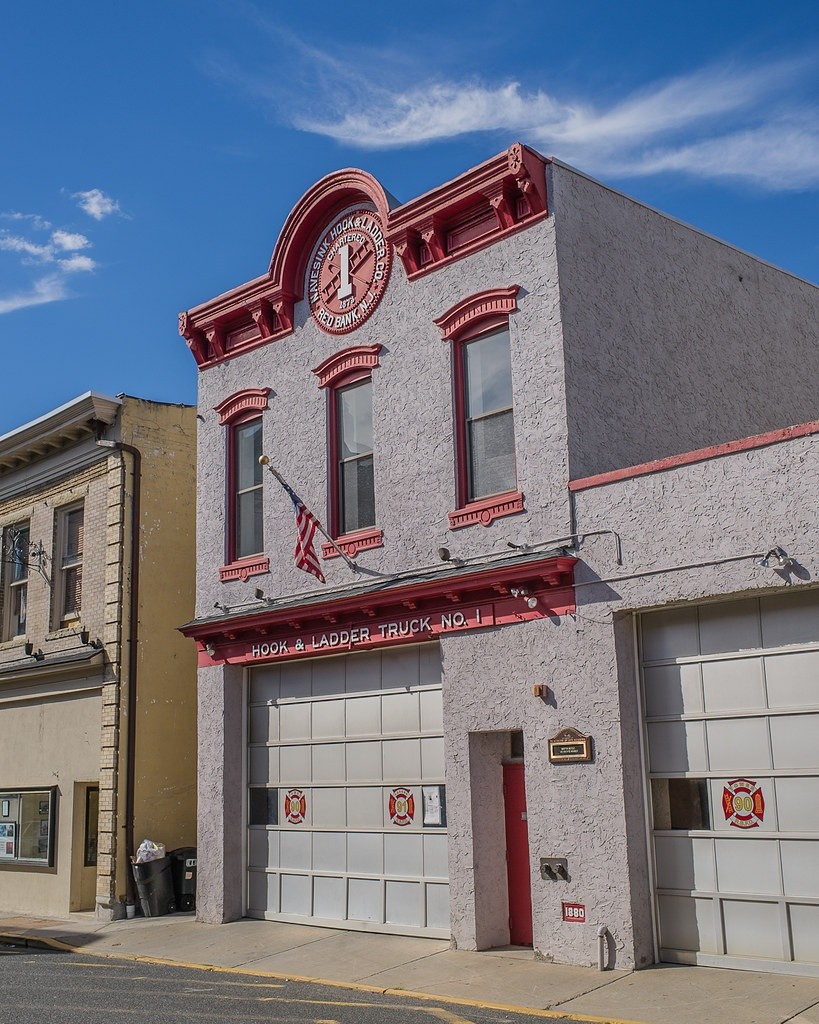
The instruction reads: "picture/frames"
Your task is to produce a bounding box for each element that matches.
[39,801,49,815]
[0,821,16,857]
[1,800,11,817]
[38,839,48,853]
[40,821,48,836]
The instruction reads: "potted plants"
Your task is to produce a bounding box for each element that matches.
[125,896,136,918]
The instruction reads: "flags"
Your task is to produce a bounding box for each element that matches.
[269,466,326,584]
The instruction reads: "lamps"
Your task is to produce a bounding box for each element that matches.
[251,589,273,604]
[80,625,100,649]
[437,546,459,563]
[25,639,42,662]
[755,544,790,568]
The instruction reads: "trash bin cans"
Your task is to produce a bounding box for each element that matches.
[165,847,197,912]
[127,839,174,918]
